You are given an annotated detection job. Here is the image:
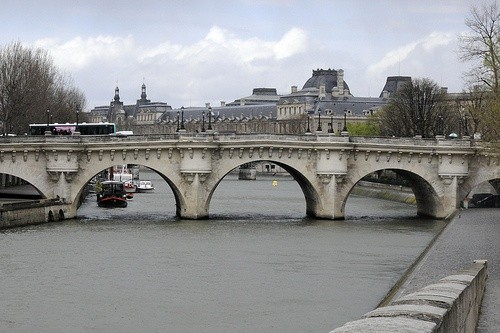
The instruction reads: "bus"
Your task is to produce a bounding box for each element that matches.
[29,121,115,137]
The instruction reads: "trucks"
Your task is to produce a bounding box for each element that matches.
[107,131,135,136]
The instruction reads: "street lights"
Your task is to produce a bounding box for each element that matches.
[316,107,324,134]
[342,109,348,134]
[175,110,181,132]
[206,107,212,132]
[329,110,334,132]
[202,110,206,133]
[305,111,312,134]
[47,107,49,131]
[179,105,186,131]
[438,115,445,134]
[74,103,80,133]
[458,118,461,137]
[465,113,468,134]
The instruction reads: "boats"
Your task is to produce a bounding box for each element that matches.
[95,180,128,209]
[138,181,156,192]
[106,167,137,199]
